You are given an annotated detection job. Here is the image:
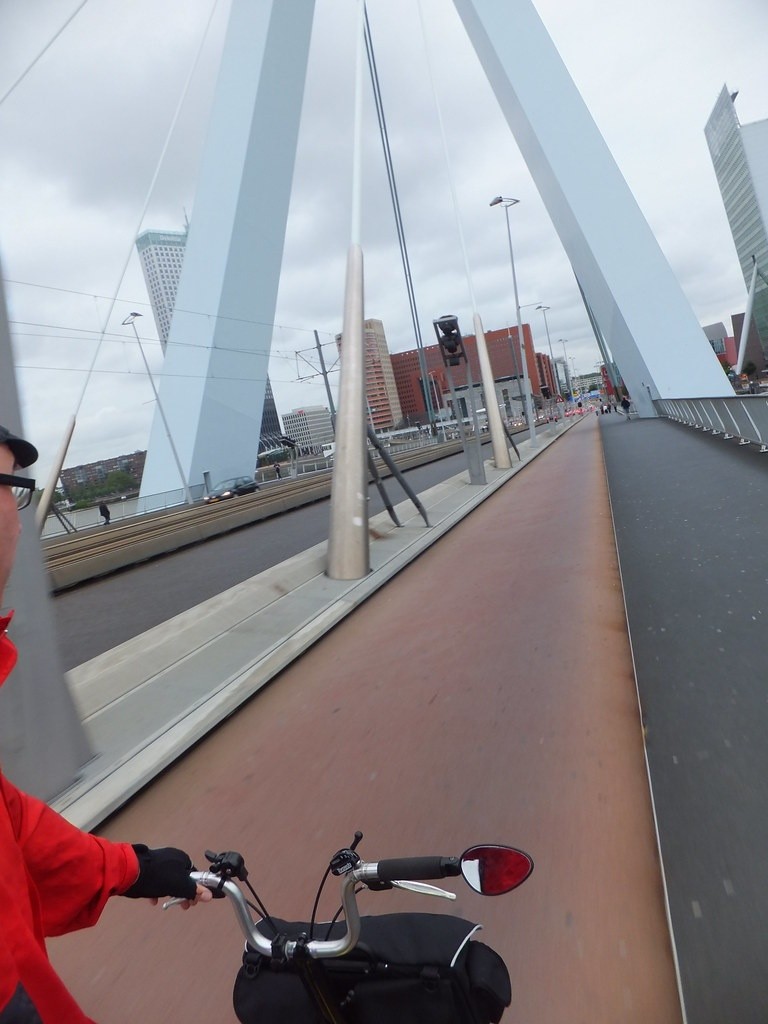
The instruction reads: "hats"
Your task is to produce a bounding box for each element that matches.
[0,422,38,468]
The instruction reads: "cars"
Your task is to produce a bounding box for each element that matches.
[203,476,261,505]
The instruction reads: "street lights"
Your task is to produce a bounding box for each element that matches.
[559,339,569,364]
[568,357,578,382]
[536,305,560,394]
[123,312,194,507]
[489,195,537,438]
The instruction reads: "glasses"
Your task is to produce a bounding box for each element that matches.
[0,469,35,510]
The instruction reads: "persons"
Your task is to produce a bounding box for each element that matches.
[273,461,281,479]
[595,402,617,417]
[0,427,213,1024]
[99,500,111,525]
[621,396,631,421]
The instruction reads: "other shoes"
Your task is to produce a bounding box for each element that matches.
[104,522,110,525]
[627,417,630,420]
